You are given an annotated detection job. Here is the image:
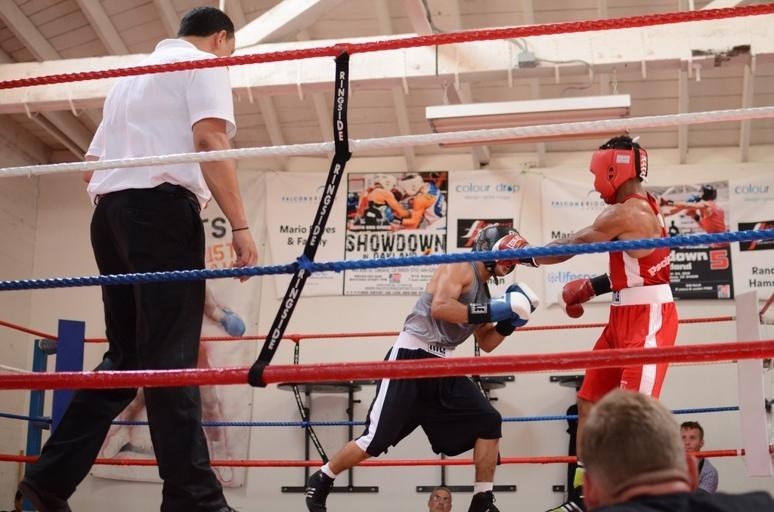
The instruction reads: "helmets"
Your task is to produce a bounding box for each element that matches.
[699,185,716,201]
[472,226,519,286]
[373,173,397,191]
[590,136,648,204]
[397,172,424,196]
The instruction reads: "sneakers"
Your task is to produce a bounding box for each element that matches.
[305,469,335,512]
[18,475,72,512]
[207,437,233,482]
[468,490,500,512]
[546,485,587,512]
[102,434,130,459]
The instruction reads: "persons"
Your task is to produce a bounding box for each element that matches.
[96,282,249,485]
[660,193,701,217]
[11,4,259,511]
[490,132,682,511]
[345,172,448,233]
[304,223,541,512]
[678,420,717,492]
[427,486,452,512]
[649,183,728,248]
[745,221,774,250]
[578,384,774,512]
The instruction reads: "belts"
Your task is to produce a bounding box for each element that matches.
[97,183,201,204]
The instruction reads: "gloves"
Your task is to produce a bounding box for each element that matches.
[496,283,540,336]
[563,273,611,318]
[491,231,539,268]
[686,194,700,203]
[221,313,245,337]
[467,291,531,327]
[685,208,700,221]
[384,206,394,222]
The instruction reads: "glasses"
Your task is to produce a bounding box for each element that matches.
[431,495,449,503]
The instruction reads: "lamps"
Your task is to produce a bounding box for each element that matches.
[427,95,634,149]
